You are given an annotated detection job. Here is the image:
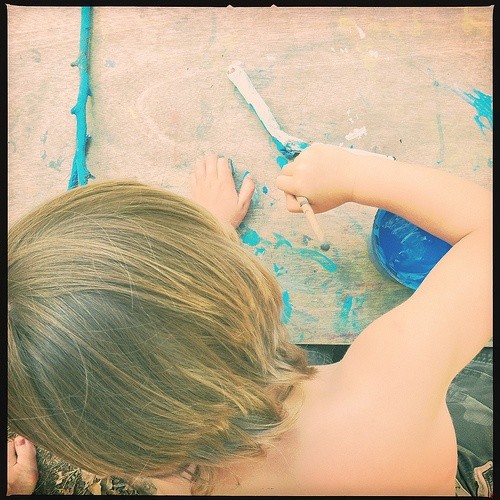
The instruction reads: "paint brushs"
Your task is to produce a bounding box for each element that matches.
[285,164,331,253]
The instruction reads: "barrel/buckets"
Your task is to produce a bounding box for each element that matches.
[368,209,453,290]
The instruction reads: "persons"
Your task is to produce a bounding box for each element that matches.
[7,139,492,497]
[8,435,39,497]
